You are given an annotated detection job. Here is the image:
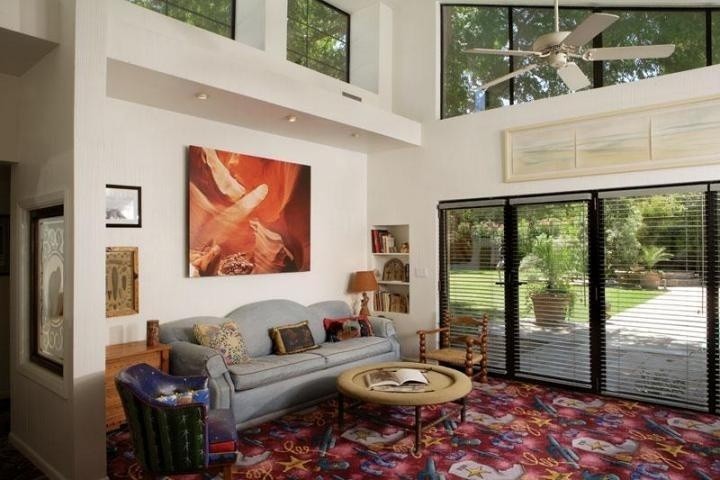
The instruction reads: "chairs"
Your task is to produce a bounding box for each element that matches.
[418,309,488,384]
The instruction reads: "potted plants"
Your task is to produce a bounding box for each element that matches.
[520,234,580,326]
[641,244,674,289]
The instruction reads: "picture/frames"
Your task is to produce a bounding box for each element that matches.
[106,247,139,318]
[105,184,143,228]
[188,145,312,278]
[505,94,720,184]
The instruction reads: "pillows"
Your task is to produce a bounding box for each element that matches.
[272,320,320,355]
[191,320,250,365]
[324,315,374,343]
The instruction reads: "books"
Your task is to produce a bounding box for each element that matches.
[371,230,397,254]
[363,369,430,390]
[376,291,402,312]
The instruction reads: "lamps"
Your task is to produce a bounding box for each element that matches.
[548,53,567,70]
[351,270,380,316]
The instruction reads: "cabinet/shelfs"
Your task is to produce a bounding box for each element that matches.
[372,226,410,313]
[105,339,171,432]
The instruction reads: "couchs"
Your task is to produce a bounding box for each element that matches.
[161,301,401,434]
[115,363,238,480]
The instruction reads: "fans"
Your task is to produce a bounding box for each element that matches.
[462,13,675,92]
